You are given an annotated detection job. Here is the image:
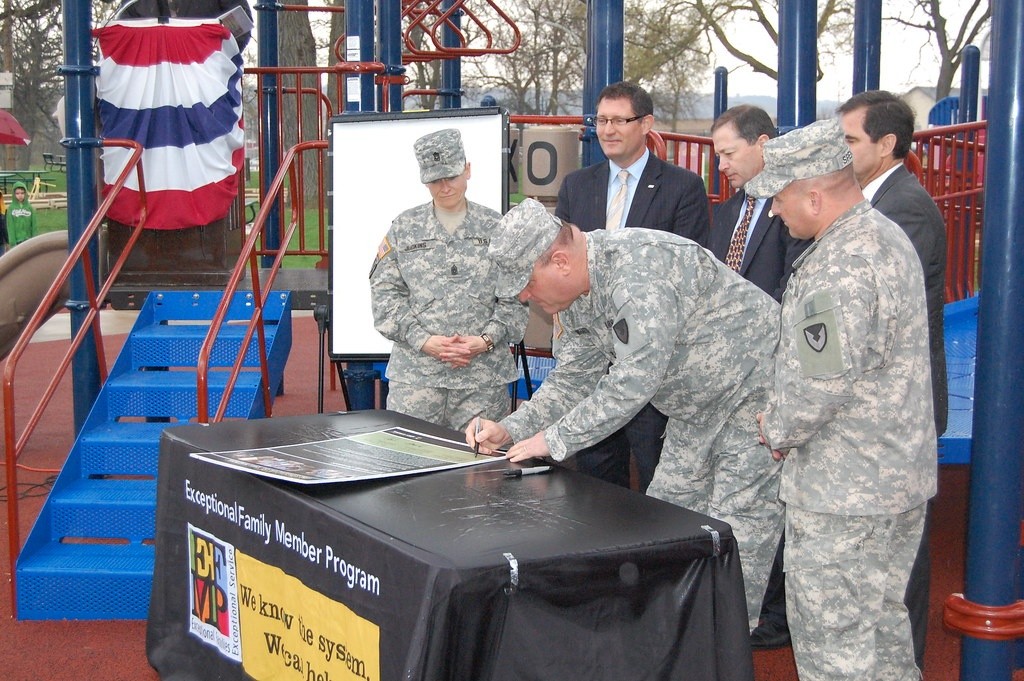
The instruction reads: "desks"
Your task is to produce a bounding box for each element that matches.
[143,406,753,681]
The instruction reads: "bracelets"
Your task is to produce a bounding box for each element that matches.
[480,333,498,355]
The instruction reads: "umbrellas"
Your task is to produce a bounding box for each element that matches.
[0,109,34,147]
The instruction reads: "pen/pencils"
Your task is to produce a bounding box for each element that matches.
[474,417,480,457]
[501,466,553,476]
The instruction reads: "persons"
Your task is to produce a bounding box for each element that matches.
[556,81,710,495]
[835,88,949,669]
[742,118,938,681]
[0,182,38,258]
[367,127,530,433]
[465,196,784,642]
[706,104,815,650]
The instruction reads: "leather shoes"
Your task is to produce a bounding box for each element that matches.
[750,621,792,649]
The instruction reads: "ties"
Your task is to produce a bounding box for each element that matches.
[606,170,629,230]
[725,195,756,273]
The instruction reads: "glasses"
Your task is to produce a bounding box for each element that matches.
[593,114,646,128]
[429,176,458,184]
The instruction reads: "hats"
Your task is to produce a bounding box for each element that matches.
[744,118,853,199]
[414,129,466,184]
[488,198,563,297]
